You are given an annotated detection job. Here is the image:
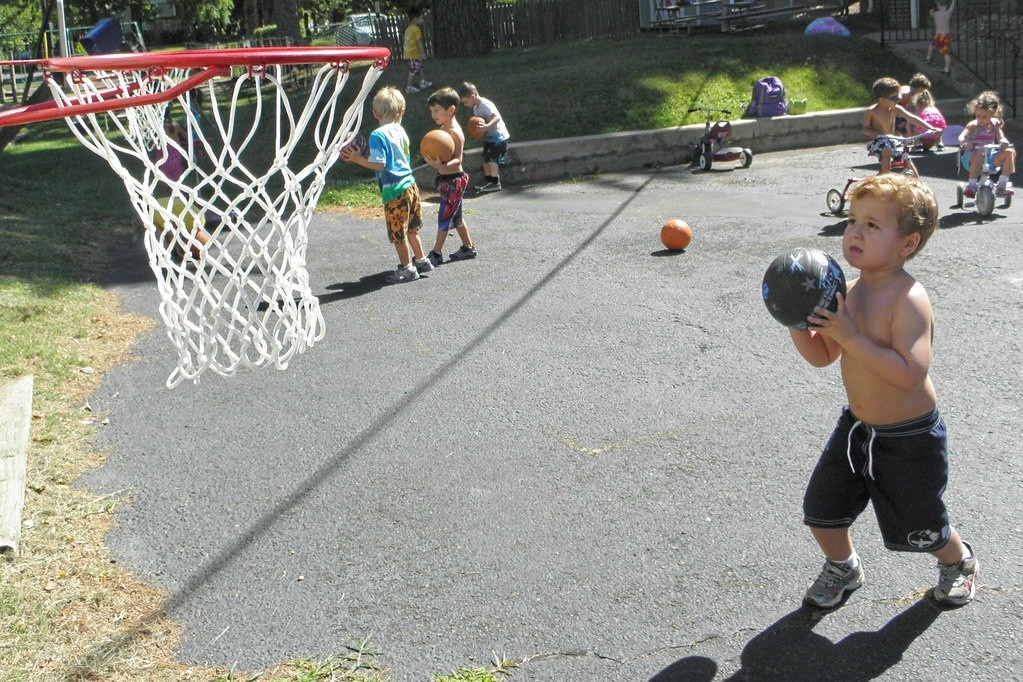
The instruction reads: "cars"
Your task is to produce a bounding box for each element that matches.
[336,13,401,47]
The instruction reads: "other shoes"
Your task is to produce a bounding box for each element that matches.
[921,57,931,64]
[938,68,950,76]
[405,86,420,95]
[419,81,432,89]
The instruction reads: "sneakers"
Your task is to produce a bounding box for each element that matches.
[934,541,979,605]
[964,184,976,198]
[449,244,477,260]
[803,555,865,608]
[411,256,434,273]
[480,182,501,192]
[427,250,443,266]
[474,181,488,189]
[386,264,420,283]
[995,185,1014,198]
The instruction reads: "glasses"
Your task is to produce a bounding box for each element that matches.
[878,94,900,103]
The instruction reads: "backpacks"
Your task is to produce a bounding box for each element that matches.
[746,76,789,117]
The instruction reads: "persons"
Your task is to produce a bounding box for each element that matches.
[862,78,942,178]
[458,81,510,192]
[895,73,947,152]
[926,0,955,76]
[402,6,433,94]
[957,91,1015,199]
[182,88,215,139]
[341,88,435,284]
[787,173,979,609]
[427,87,477,267]
[150,101,206,253]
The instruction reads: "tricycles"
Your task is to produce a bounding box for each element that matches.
[955,144,1014,218]
[688,107,753,171]
[825,129,939,212]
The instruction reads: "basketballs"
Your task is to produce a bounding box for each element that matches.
[661,219,691,250]
[762,246,846,331]
[180,230,209,258]
[467,116,488,137]
[420,130,455,164]
[340,131,367,164]
